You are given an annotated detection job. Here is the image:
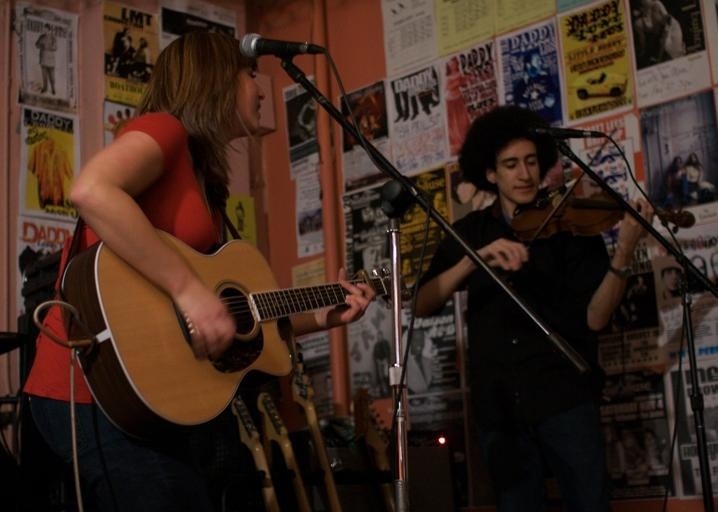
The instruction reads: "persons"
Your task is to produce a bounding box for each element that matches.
[235,199,245,231]
[28,137,74,210]
[389,67,441,121]
[35,23,58,97]
[666,155,683,205]
[22,30,377,512]
[661,251,718,296]
[406,105,661,511]
[511,50,558,127]
[679,152,718,201]
[374,330,392,387]
[404,327,435,392]
[111,27,153,80]
[602,424,673,501]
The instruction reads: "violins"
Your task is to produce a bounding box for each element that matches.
[511,184,694,241]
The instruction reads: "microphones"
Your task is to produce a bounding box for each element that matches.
[532,124,606,142]
[240,33,323,59]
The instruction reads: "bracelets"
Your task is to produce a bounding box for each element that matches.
[607,263,635,281]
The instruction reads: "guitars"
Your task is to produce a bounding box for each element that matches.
[230,330,401,512]
[59,226,411,442]
